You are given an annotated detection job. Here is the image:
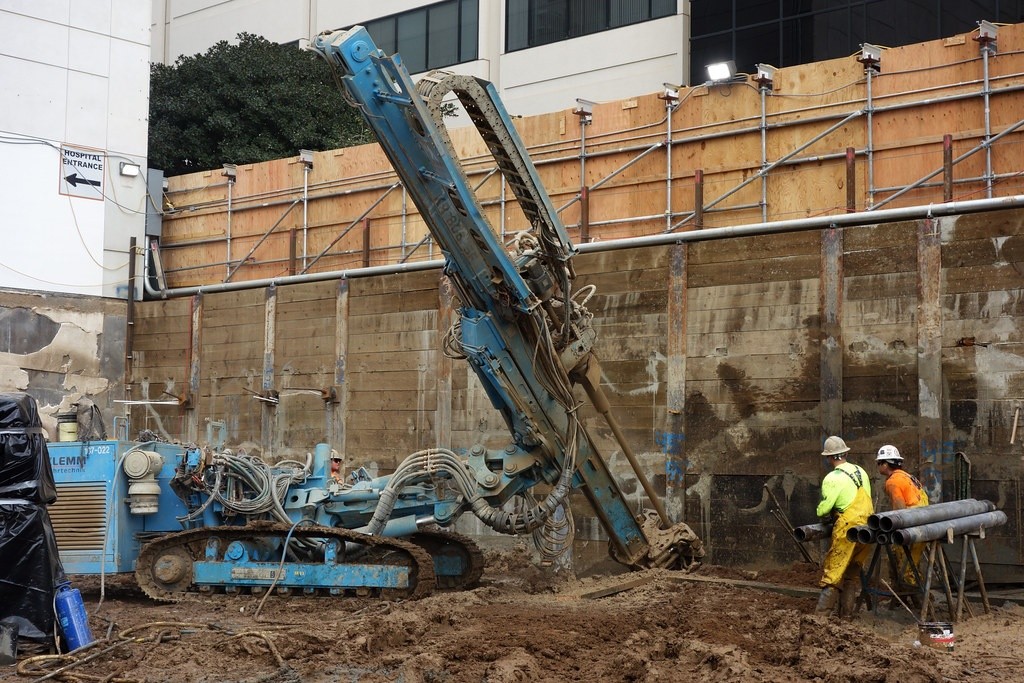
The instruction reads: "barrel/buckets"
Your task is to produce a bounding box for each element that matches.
[59,422,78,441]
[918,621,954,654]
[57,414,77,441]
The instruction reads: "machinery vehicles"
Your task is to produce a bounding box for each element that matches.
[46,25,708,611]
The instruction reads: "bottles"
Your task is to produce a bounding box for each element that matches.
[53,580,93,651]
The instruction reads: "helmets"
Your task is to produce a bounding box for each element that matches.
[821,436,851,456]
[874,445,904,461]
[331,449,342,459]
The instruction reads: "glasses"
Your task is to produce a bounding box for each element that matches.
[333,459,341,463]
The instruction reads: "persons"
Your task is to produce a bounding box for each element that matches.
[816,437,879,615]
[875,444,930,613]
[330,449,345,483]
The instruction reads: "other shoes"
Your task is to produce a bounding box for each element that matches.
[911,586,927,609]
[812,590,838,618]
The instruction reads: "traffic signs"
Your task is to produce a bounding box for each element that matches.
[54,140,107,202]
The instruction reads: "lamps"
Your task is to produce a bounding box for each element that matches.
[707,60,736,82]
[120,162,141,177]
